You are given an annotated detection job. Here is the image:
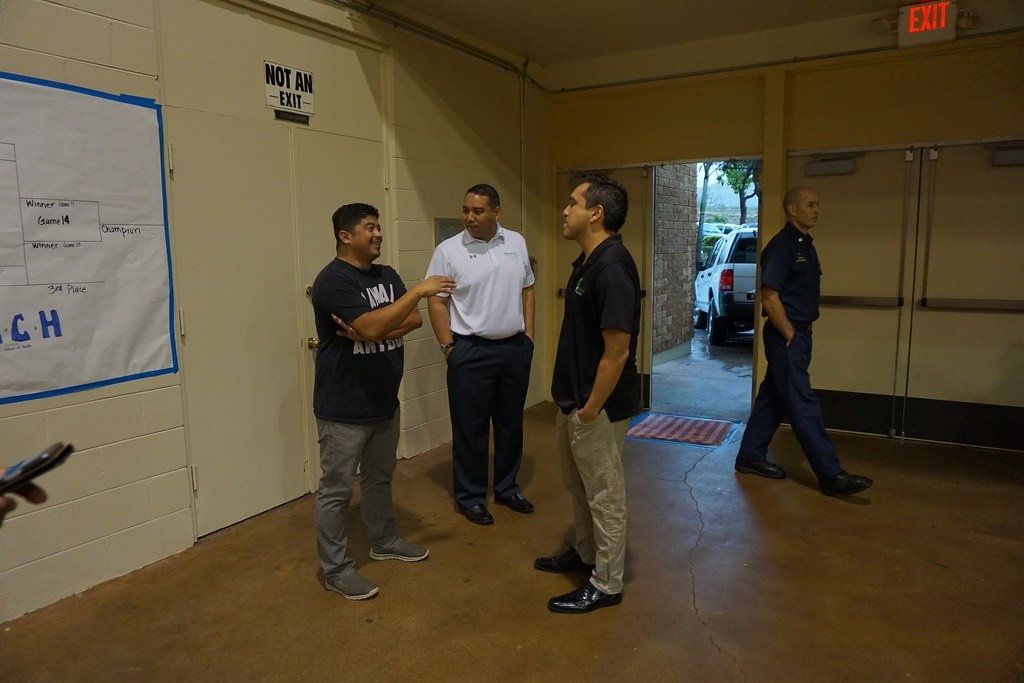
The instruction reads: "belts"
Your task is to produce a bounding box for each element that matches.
[792,321,814,331]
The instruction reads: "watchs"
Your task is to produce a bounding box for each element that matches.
[441,343,455,353]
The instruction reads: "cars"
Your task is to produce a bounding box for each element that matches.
[695,220,758,236]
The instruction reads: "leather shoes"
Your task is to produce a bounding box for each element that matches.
[548,581,622,613]
[735,462,786,479]
[821,475,873,496]
[459,503,493,524]
[533,540,595,579]
[496,491,534,512]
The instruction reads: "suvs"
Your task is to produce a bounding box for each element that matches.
[692,227,759,346]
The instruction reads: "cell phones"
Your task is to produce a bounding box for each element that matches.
[0,440,62,486]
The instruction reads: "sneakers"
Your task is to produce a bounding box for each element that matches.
[369,534,429,561]
[324,569,379,599]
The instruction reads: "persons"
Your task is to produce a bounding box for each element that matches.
[735,188,873,496]
[425,184,535,525]
[535,177,642,614]
[313,203,456,599]
